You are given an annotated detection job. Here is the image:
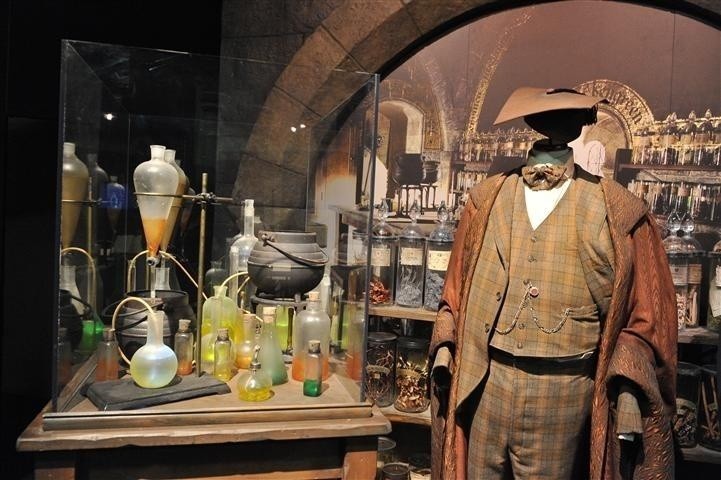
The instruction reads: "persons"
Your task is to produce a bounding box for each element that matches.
[429,84,676,480]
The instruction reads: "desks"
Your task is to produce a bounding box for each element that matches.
[16,348,392,480]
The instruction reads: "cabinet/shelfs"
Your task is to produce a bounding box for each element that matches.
[327,148,721,480]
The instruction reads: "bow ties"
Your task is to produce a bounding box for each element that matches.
[521,163,569,192]
